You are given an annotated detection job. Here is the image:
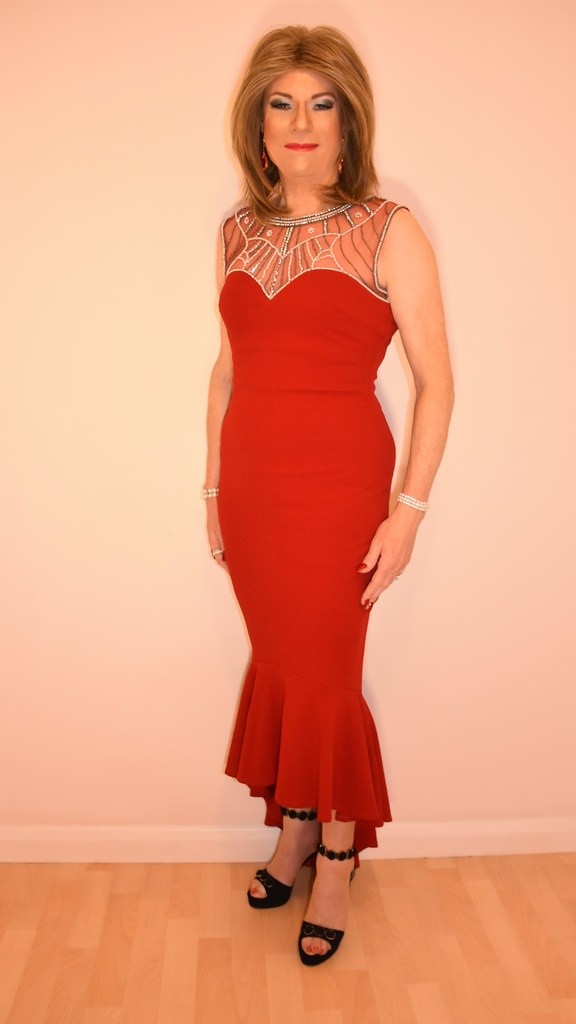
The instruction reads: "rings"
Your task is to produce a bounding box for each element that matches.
[211,547,222,559]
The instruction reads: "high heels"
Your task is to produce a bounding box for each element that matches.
[298,843,358,964]
[248,807,319,908]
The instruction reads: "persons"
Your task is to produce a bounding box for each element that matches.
[202,20,459,968]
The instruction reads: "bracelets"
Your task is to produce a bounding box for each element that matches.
[203,488,219,499]
[396,493,432,511]
